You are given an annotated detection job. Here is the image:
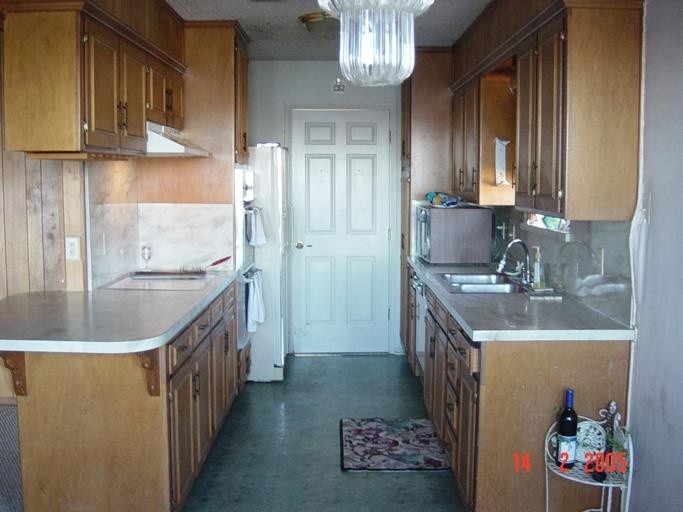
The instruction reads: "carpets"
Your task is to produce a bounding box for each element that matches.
[340,417,452,474]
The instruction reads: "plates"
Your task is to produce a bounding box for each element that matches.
[576,420,606,463]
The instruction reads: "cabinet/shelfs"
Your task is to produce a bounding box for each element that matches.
[442,316,626,512]
[145,1,185,130]
[514,4,642,221]
[544,413,634,511]
[17,308,213,512]
[1,0,145,160]
[238,342,250,391]
[212,285,238,437]
[406,271,417,375]
[451,67,516,207]
[423,288,449,440]
[139,21,249,204]
[400,48,451,352]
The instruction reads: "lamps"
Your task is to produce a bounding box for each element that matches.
[299,12,342,41]
[318,0,434,88]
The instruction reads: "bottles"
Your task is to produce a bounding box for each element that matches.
[556,389,576,469]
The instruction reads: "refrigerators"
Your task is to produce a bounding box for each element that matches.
[244,143,289,382]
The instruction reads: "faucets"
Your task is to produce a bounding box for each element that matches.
[494,236,534,282]
[555,238,598,283]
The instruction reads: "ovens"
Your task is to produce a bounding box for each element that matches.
[234,169,263,273]
[238,267,263,345]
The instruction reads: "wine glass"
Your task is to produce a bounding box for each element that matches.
[141,246,153,270]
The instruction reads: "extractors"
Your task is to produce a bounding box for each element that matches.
[145,121,209,160]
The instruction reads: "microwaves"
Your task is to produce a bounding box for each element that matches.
[415,204,492,265]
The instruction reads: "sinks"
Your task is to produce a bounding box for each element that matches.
[443,283,532,295]
[435,266,532,282]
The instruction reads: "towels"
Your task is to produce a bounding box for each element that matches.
[247,270,266,334]
[246,208,266,246]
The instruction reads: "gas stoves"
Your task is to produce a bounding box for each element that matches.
[130,267,208,280]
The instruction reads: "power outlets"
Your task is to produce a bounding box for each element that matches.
[64,237,81,261]
